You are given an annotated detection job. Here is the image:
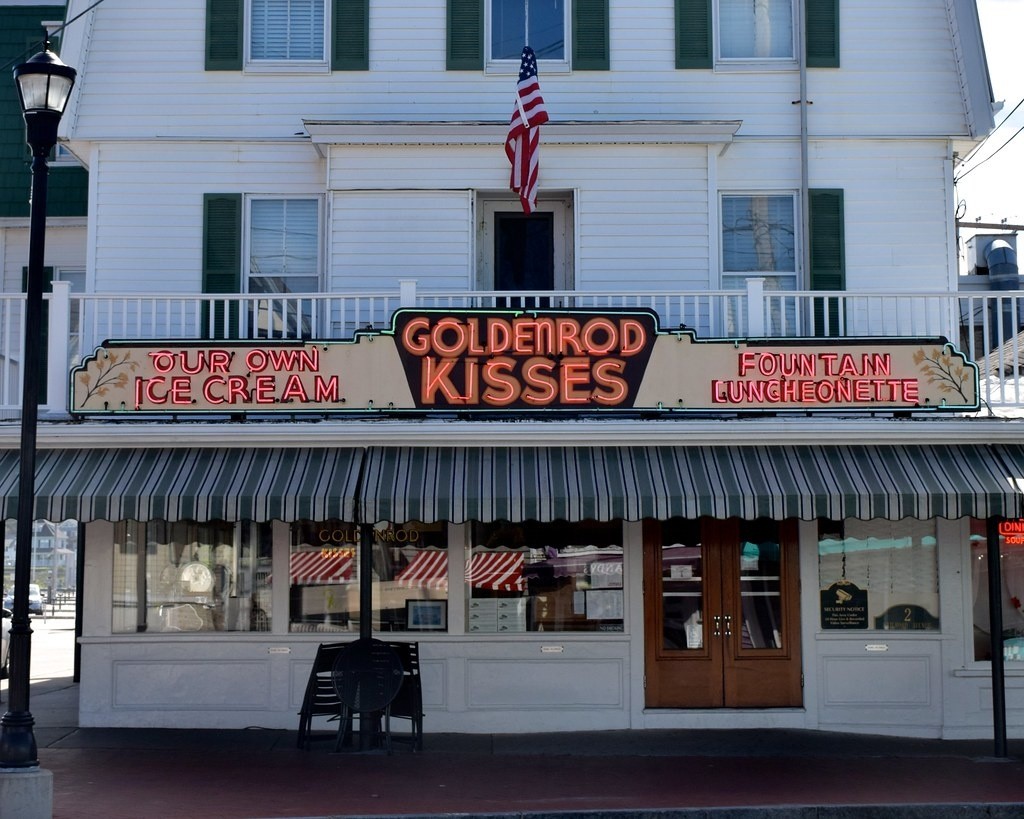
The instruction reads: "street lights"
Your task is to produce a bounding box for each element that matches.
[0,39,80,768]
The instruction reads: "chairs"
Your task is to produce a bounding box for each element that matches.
[296,636,424,757]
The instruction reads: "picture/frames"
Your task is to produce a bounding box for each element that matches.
[405,599,448,631]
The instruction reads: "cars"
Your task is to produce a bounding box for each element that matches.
[2,584,46,615]
[2,608,13,678]
